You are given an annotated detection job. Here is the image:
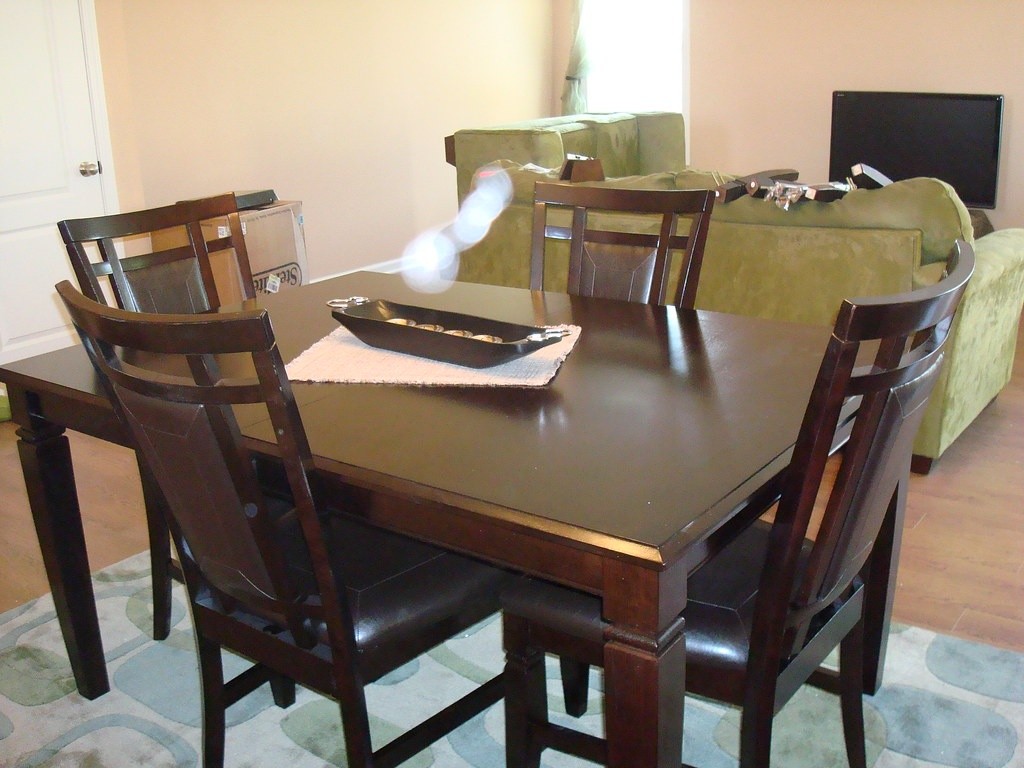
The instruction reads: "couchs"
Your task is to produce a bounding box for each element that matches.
[445,109,1024,476]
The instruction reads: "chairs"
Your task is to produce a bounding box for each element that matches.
[55,280,510,768]
[58,193,299,712]
[499,238,973,768]
[526,183,723,306]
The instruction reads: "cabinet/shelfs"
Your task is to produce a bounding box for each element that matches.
[148,202,306,312]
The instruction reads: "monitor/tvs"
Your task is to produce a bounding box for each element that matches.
[828,91,1003,210]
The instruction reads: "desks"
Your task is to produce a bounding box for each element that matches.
[0,271,928,768]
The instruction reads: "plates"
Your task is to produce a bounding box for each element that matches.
[330,298,571,368]
